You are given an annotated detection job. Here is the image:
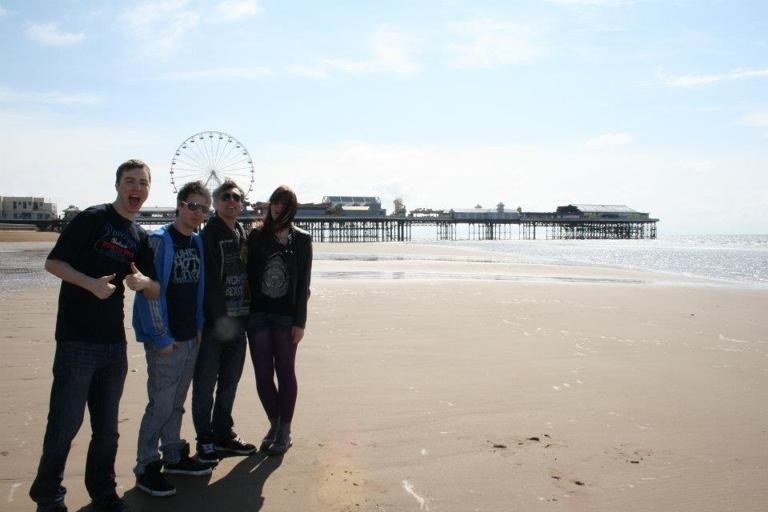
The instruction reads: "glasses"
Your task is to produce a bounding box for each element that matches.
[180,200,208,213]
[221,193,240,201]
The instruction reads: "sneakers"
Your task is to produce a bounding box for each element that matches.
[135,475,176,497]
[262,429,292,455]
[164,458,211,476]
[196,435,256,463]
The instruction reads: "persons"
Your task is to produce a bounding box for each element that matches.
[192,179,264,464]
[242,184,313,456]
[28,158,161,512]
[131,180,212,497]
